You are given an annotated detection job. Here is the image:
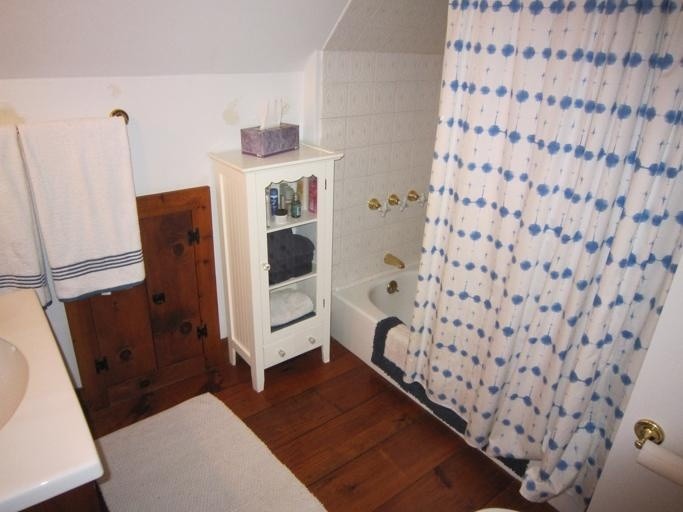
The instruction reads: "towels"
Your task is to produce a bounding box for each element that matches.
[266,290,316,327]
[268,232,313,284]
[371,318,534,480]
[0,115,148,310]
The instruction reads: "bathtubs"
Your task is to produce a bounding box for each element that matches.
[331,263,602,512]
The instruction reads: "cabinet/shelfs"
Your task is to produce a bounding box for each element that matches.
[208,143,344,394]
[63,185,225,418]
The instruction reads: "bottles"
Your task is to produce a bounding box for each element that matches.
[269,174,316,218]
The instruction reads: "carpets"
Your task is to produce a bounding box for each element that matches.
[93,391,331,511]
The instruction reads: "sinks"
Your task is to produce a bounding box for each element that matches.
[0,337,29,432]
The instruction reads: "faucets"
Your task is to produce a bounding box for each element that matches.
[384,253,404,270]
